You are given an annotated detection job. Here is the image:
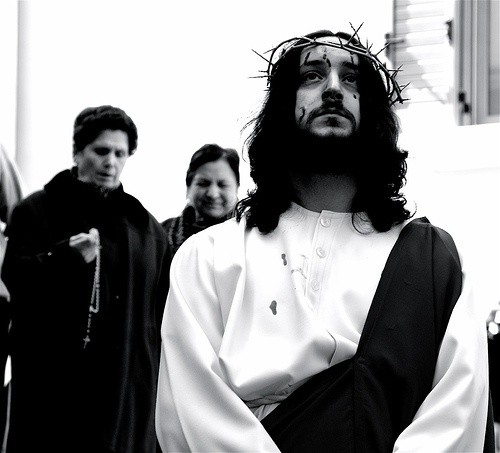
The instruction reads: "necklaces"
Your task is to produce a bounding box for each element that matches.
[83,233,101,348]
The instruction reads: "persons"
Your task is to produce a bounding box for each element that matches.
[155,22,495,453]
[159,144,248,254]
[0,105,178,453]
